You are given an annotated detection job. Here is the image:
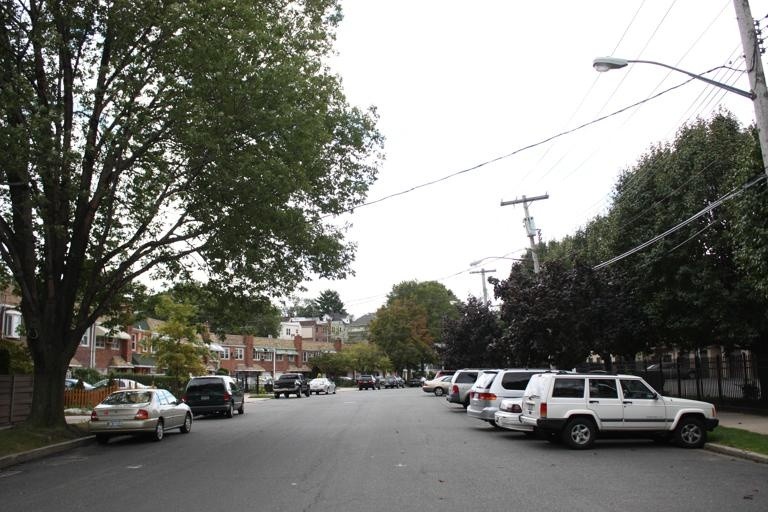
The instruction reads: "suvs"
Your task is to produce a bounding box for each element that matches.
[422,368,722,452]
[181,375,245,419]
[356,373,427,390]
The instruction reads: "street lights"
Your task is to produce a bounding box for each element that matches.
[591,54,768,177]
[469,256,543,273]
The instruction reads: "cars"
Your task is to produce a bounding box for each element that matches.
[65,379,96,392]
[642,363,700,382]
[306,377,337,395]
[88,388,194,442]
[340,375,353,381]
[91,378,158,390]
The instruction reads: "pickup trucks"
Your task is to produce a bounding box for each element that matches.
[271,372,311,399]
